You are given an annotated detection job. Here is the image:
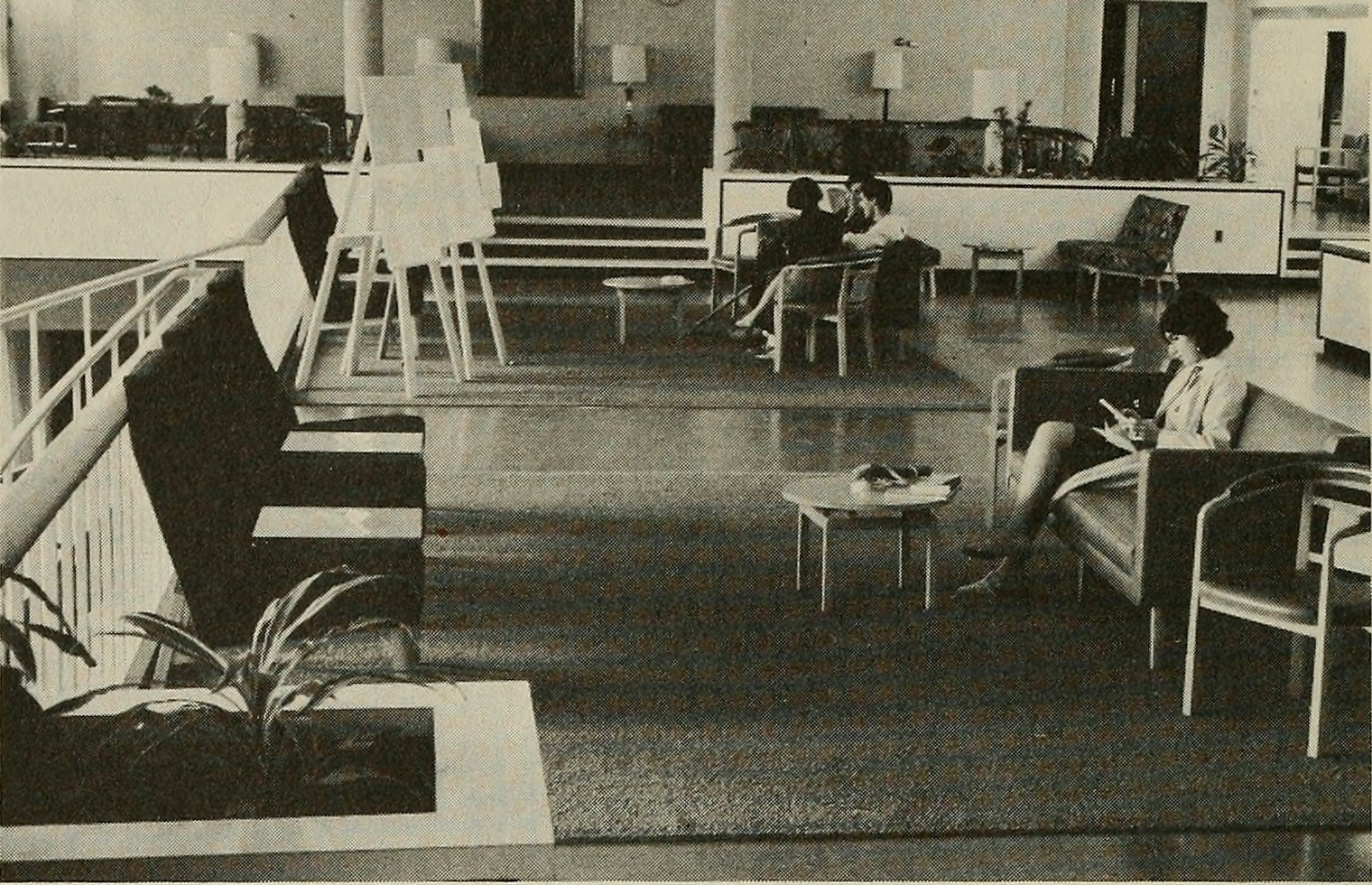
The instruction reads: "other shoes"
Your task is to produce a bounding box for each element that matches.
[963,528,1034,560]
[956,572,1030,601]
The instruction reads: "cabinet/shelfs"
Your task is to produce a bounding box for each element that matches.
[1318,239,1371,370]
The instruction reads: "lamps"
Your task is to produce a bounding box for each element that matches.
[611,42,646,132]
[971,69,1024,178]
[870,50,902,122]
[418,36,454,64]
[208,30,262,161]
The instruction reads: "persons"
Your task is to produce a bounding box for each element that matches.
[948,293,1248,614]
[833,177,907,256]
[830,171,877,233]
[735,174,842,365]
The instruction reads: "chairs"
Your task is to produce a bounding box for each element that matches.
[1057,195,1190,316]
[122,265,429,681]
[769,230,916,378]
[709,209,802,322]
[986,343,1136,527]
[286,163,428,362]
[1292,132,1369,215]
[1182,458,1372,758]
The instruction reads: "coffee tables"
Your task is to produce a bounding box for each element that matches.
[604,275,694,346]
[779,476,956,611]
[961,240,1034,300]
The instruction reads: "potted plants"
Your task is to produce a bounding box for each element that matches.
[2,555,555,881]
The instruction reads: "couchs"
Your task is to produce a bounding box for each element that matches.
[1005,366,1372,669]
